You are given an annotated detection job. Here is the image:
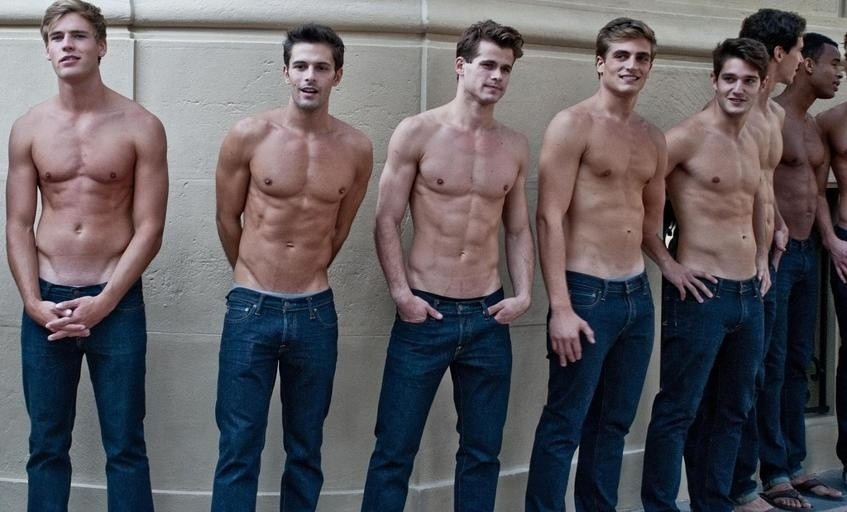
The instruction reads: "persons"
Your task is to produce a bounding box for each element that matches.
[699,9,807,512]
[640,37,773,511]
[359,20,534,512]
[5,0,168,512]
[523,16,669,512]
[758,34,843,512]
[209,26,374,512]
[814,38,847,465]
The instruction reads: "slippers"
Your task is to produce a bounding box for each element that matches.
[759,489,815,511]
[795,478,843,502]
